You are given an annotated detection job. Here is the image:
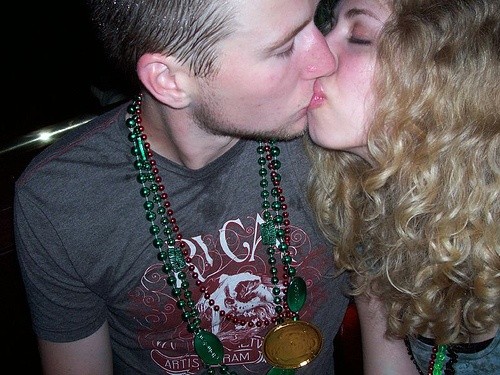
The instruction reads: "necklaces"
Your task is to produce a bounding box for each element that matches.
[402,335,458,375]
[127,90,322,375]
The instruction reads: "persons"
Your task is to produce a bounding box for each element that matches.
[15,0,353,375]
[302,0,500,375]
